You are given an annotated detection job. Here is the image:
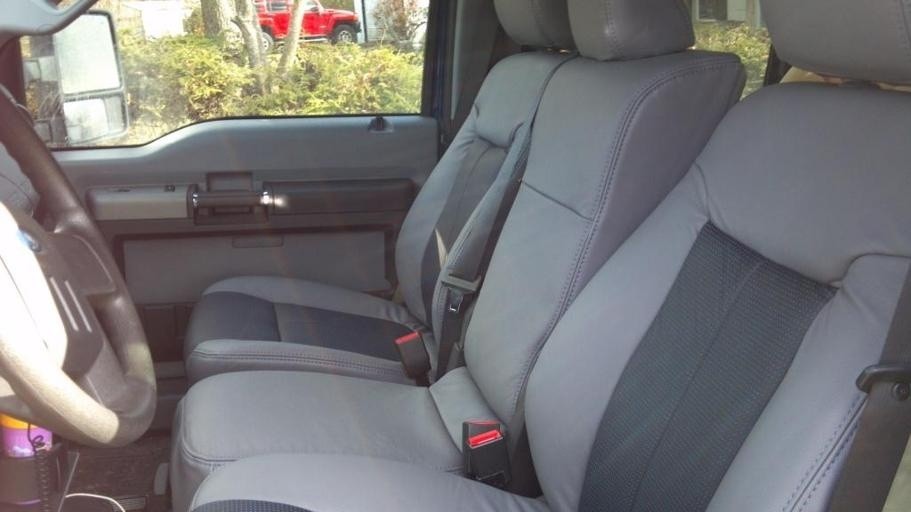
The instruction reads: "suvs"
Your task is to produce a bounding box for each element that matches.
[254,0,361,53]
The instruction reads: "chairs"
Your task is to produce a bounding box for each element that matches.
[170,1,750,512]
[189,2,911,511]
[179,1,573,384]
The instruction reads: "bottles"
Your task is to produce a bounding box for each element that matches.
[1,394,60,506]
